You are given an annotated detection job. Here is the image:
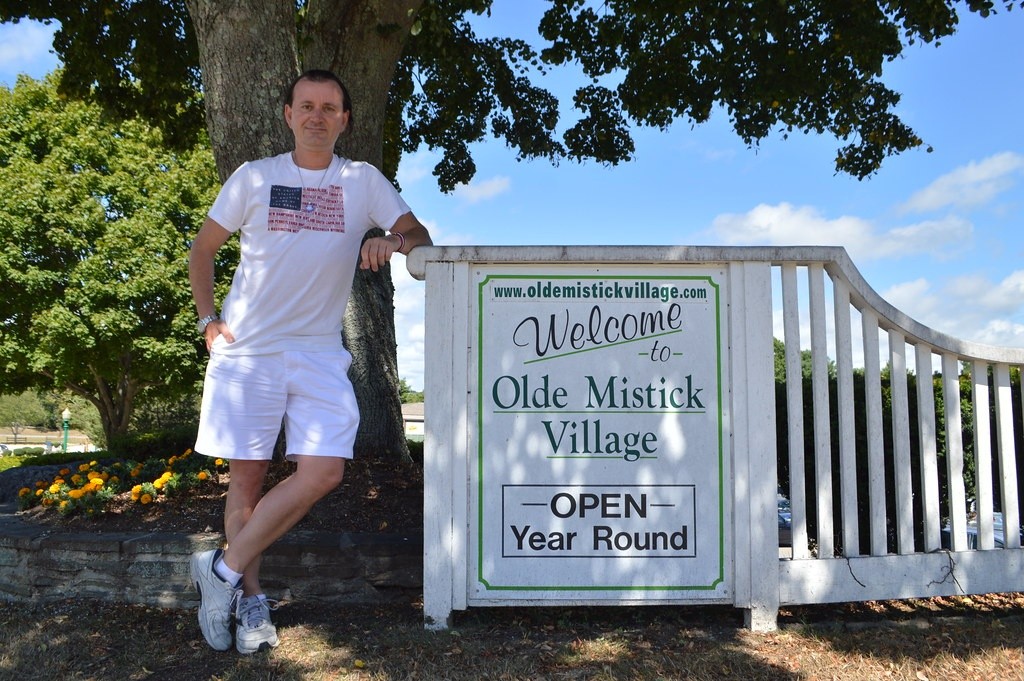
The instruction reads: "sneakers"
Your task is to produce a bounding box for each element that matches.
[189,549,244,651]
[236,595,282,655]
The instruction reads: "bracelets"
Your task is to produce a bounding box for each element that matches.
[392,231,405,252]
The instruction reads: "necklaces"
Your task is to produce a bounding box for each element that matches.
[293,151,330,212]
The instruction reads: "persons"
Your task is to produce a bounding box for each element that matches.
[189,69,435,654]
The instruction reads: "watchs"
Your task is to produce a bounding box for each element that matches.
[196,314,218,334]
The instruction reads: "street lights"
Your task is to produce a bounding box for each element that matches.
[61,407,72,454]
